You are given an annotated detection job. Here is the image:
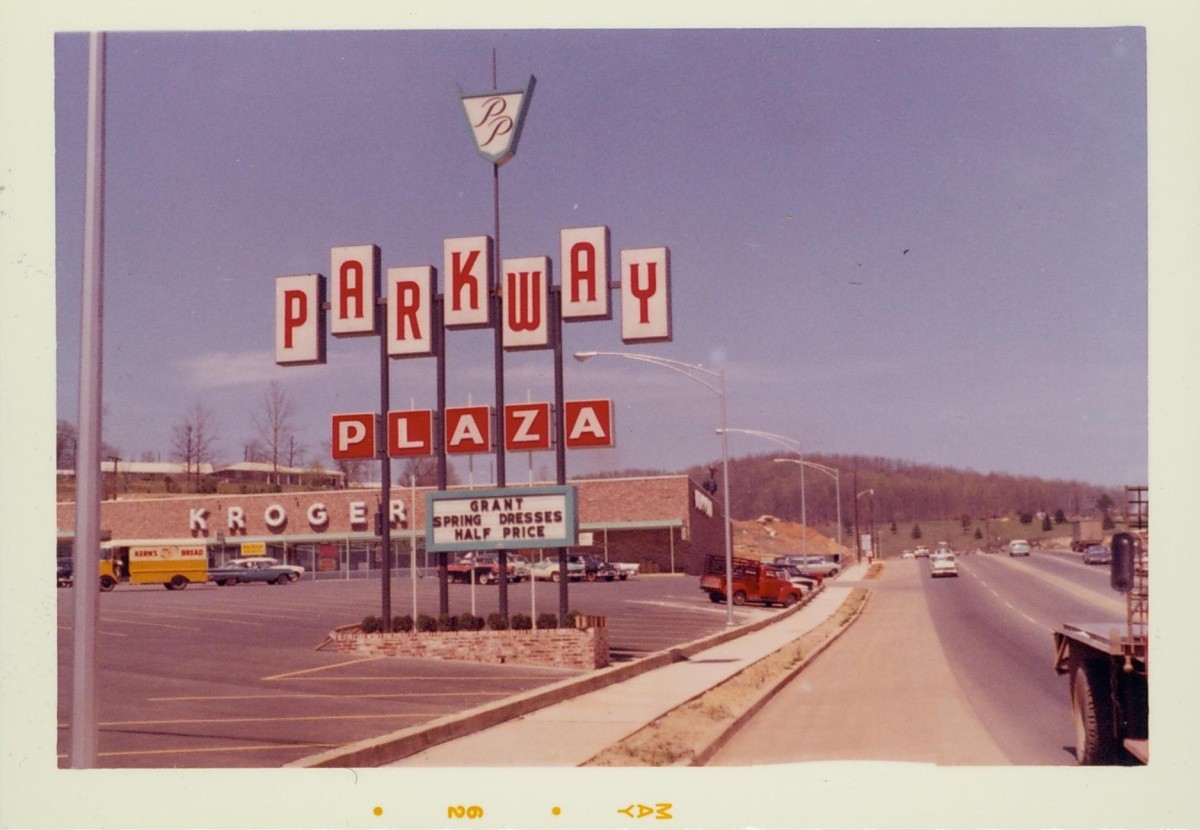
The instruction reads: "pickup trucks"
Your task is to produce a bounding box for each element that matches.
[701,553,843,609]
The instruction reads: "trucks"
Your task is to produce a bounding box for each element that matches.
[98,541,209,593]
[1051,487,1149,765]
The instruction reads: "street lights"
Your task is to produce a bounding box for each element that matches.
[712,426,809,579]
[770,458,843,561]
[853,471,875,564]
[573,351,735,628]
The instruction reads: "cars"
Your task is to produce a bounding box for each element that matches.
[1082,544,1112,565]
[900,549,916,559]
[435,551,637,586]
[913,545,930,558]
[1008,538,1032,557]
[57,556,74,588]
[209,556,306,587]
[928,539,961,577]
